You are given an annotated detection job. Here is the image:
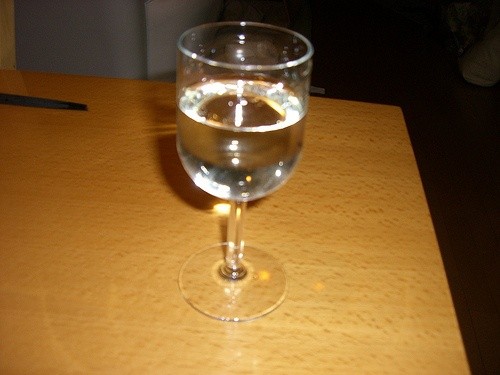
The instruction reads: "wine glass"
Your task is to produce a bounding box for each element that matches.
[175,22,314,322]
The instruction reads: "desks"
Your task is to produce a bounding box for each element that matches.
[0,69,469,375]
